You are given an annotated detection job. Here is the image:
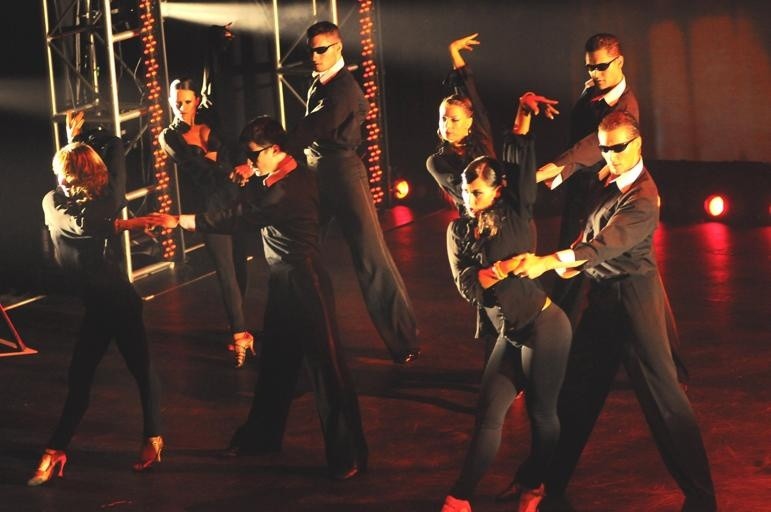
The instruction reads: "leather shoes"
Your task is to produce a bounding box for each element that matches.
[397,352,421,367]
[332,463,359,482]
[214,444,245,460]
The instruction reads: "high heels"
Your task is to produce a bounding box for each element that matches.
[27,452,67,486]
[134,437,164,474]
[231,332,256,371]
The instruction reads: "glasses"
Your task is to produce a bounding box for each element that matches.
[245,144,273,161]
[585,59,616,72]
[597,138,634,152]
[305,43,337,55]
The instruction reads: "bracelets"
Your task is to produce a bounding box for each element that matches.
[518,91,537,111]
[492,261,507,281]
[176,215,178,226]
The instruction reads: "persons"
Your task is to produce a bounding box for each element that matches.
[229,21,423,386]
[156,17,257,368]
[438,91,573,512]
[145,117,369,482]
[535,33,691,398]
[27,107,162,486]
[424,33,496,376]
[510,110,718,512]
